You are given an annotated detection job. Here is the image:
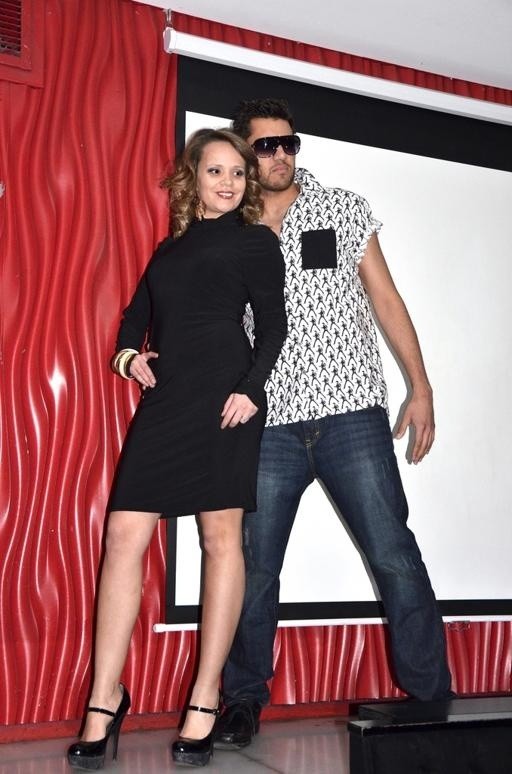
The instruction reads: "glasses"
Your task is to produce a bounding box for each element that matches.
[248,135,301,158]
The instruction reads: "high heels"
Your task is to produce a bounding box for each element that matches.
[171,687,221,766]
[67,682,131,770]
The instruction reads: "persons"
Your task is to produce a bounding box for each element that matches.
[60,123,293,773]
[206,92,460,755]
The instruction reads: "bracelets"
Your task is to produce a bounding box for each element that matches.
[109,348,140,381]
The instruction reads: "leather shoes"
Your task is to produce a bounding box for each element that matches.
[212,702,262,750]
